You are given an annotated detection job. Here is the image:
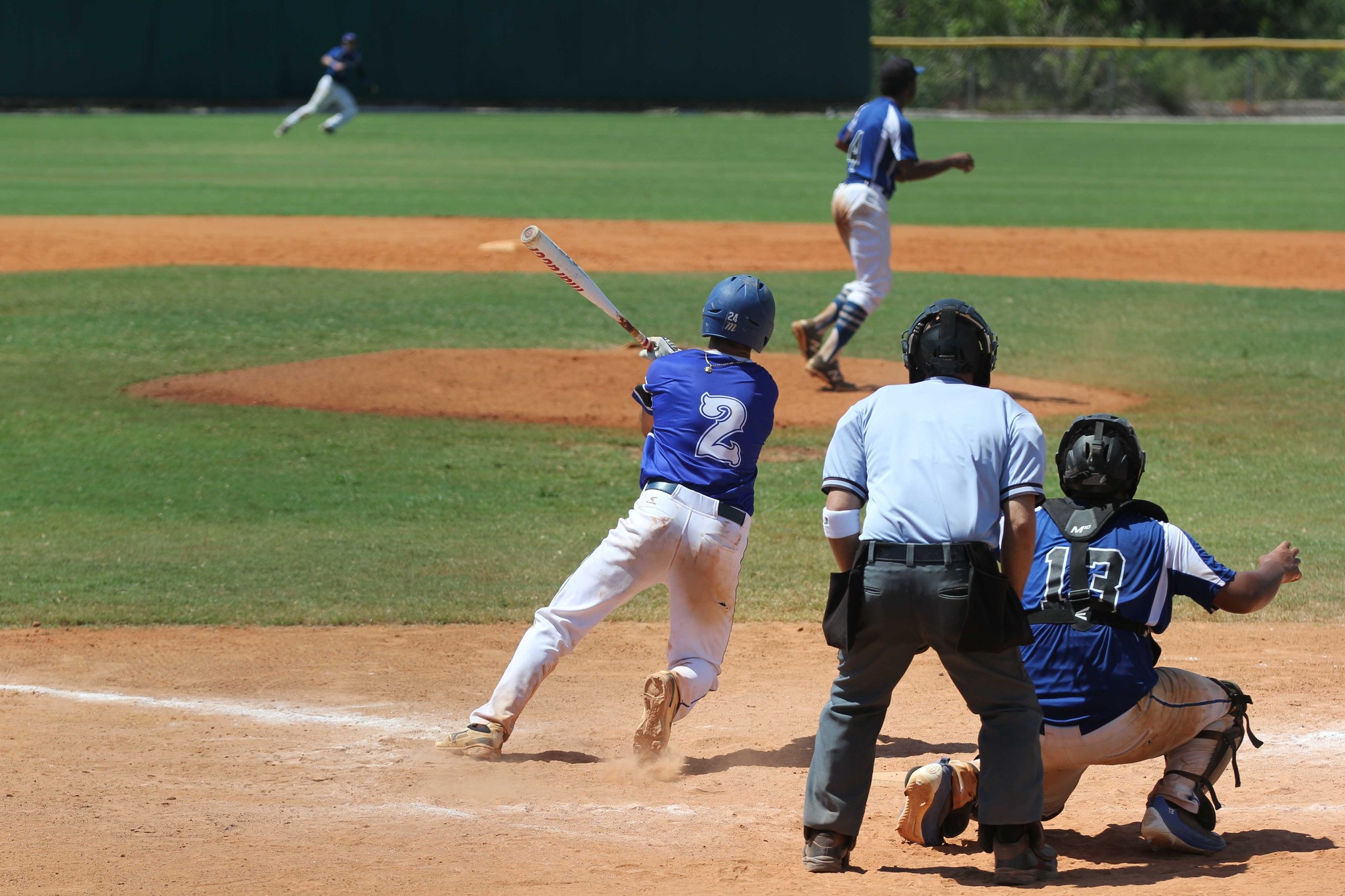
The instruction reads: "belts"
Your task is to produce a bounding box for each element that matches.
[861,540,985,564]
[646,479,746,526]
[1036,694,1141,736]
[865,179,885,194]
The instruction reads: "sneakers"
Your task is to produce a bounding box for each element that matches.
[896,757,954,844]
[436,723,505,763]
[802,834,849,872]
[995,834,1059,886]
[1140,797,1223,853]
[792,319,823,360]
[634,672,681,762]
[805,355,855,391]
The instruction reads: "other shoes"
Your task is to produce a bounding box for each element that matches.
[318,124,334,134]
[275,123,288,138]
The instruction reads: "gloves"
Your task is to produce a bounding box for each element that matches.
[639,337,683,361]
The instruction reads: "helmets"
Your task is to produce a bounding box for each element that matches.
[1056,414,1145,501]
[1061,427,1137,498]
[702,275,775,353]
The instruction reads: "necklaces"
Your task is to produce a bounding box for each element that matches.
[704,351,751,373]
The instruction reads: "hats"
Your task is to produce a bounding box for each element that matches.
[881,57,924,92]
[917,314,982,371]
[344,33,356,42]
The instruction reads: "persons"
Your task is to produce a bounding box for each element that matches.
[897,412,1302,854]
[791,57,974,392]
[802,297,1058,887]
[273,32,359,140]
[435,274,779,759]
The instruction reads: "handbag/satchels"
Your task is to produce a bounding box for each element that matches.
[822,546,868,653]
[962,542,1033,649]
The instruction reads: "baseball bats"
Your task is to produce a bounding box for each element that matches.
[520,227,664,358]
